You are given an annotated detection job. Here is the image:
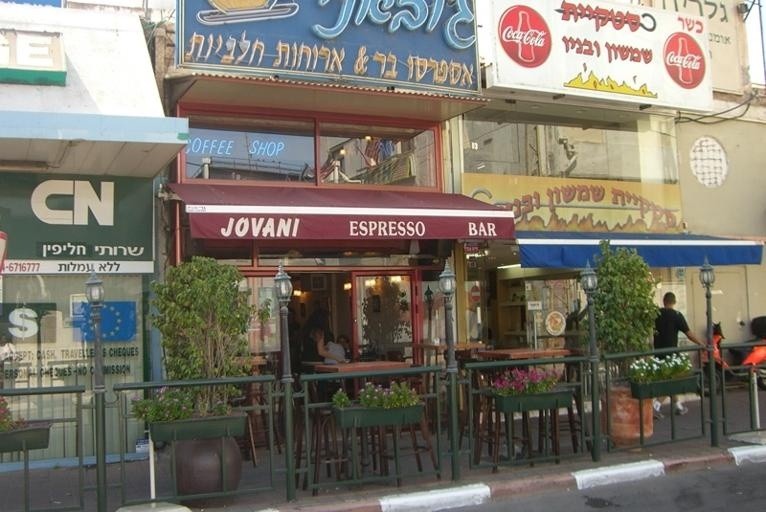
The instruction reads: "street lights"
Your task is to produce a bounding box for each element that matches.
[439,260,459,480]
[274,262,296,500]
[580,262,600,461]
[424,282,435,369]
[699,256,720,447]
[85,269,107,512]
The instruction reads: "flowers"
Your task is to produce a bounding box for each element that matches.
[628,352,693,381]
[332,382,419,411]
[489,366,563,394]
[0,396,15,432]
[129,392,194,421]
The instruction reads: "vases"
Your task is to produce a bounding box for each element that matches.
[149,416,249,442]
[493,395,572,410]
[0,428,49,454]
[333,404,425,429]
[630,376,698,399]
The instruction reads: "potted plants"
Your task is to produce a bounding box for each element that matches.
[582,237,662,446]
[152,258,256,508]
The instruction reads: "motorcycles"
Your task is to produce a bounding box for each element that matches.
[693,316,766,396]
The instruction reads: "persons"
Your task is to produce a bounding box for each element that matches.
[653,292,705,419]
[293,308,350,402]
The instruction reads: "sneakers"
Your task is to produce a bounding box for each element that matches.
[653,408,665,419]
[675,407,689,416]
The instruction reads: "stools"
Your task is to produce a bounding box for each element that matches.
[229,342,593,497]
